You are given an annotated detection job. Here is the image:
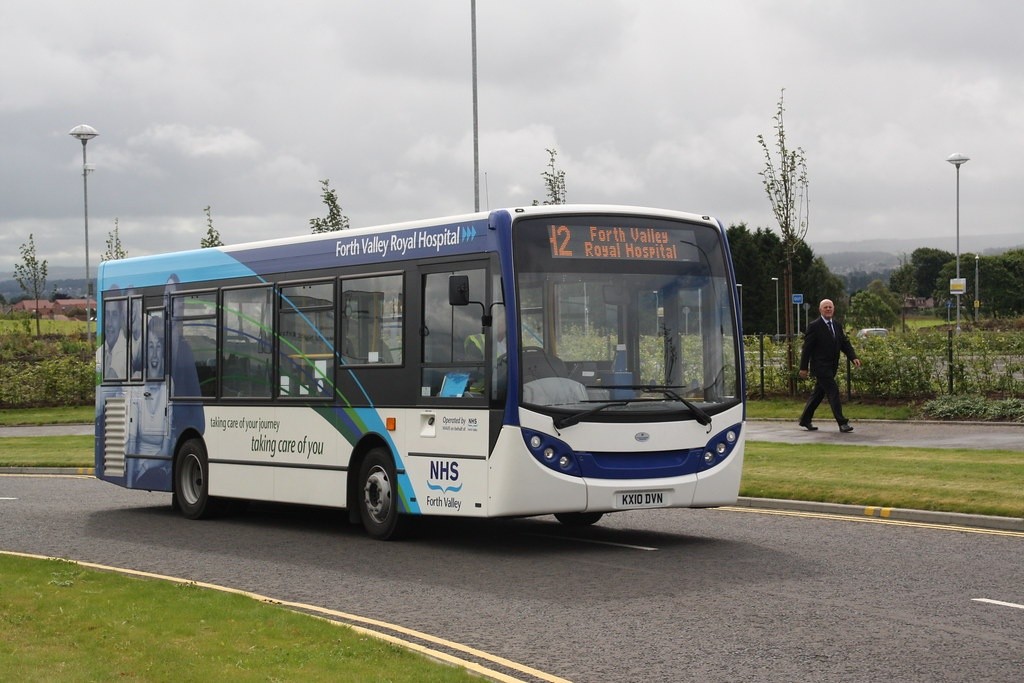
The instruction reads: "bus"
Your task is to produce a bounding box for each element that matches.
[93,205,747,538]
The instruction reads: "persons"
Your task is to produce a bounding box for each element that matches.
[95,273,205,490]
[799,299,860,433]
[453,304,506,392]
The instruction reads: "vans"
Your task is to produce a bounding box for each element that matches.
[856,328,889,338]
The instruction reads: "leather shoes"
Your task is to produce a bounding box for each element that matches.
[840,424,853,432]
[799,420,818,430]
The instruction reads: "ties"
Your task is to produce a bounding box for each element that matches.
[827,321,833,335]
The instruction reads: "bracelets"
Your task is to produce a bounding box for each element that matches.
[141,464,147,472]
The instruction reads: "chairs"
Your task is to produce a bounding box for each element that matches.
[497,346,568,400]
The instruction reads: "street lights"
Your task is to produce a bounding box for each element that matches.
[735,284,743,334]
[653,290,659,334]
[975,251,979,321]
[68,125,99,346]
[944,152,972,335]
[771,276,780,344]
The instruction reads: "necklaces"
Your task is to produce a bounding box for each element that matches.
[150,383,161,391]
